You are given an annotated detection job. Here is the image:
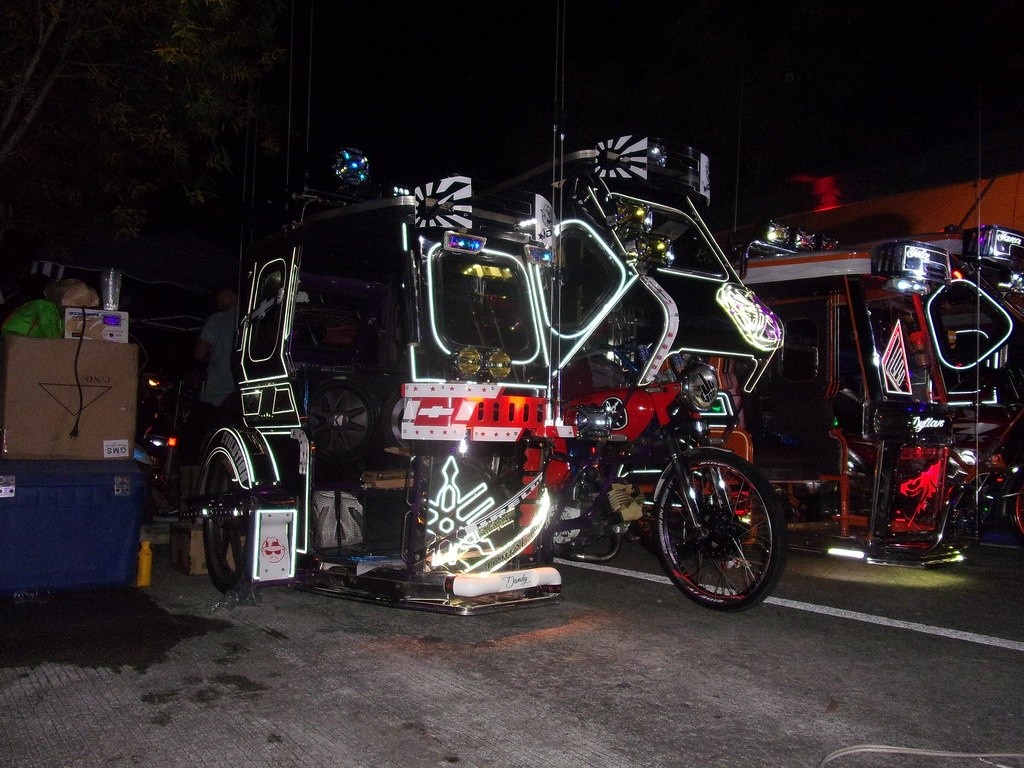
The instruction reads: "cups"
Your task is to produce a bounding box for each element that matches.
[101,268,122,311]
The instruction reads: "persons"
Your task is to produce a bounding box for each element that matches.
[184,282,245,469]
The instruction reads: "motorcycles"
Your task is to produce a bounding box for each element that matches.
[725,218,1024,568]
[174,132,786,615]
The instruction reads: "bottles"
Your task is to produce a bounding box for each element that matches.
[136,541,152,586]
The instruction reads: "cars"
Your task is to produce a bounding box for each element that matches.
[138,413,205,519]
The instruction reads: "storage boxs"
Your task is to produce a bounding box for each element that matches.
[168,521,236,575]
[0,306,149,595]
